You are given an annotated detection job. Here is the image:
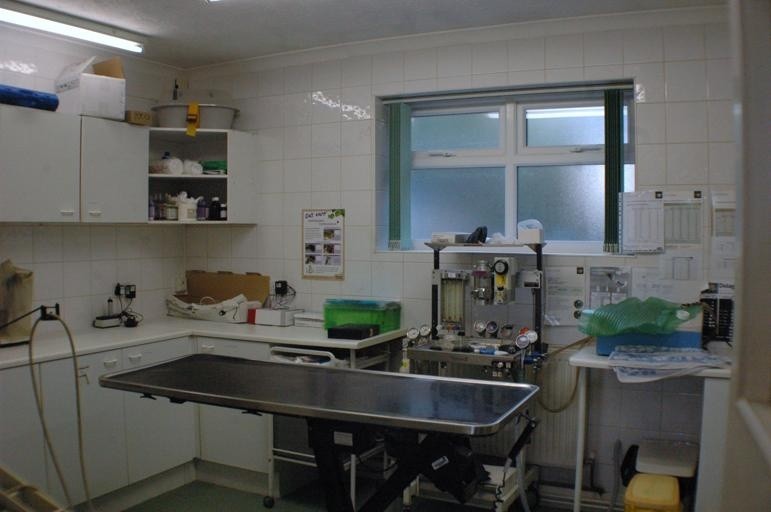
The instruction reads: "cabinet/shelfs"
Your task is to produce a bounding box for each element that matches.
[1,361,52,506]
[197,336,392,512]
[0,103,148,224]
[145,126,257,225]
[39,336,195,512]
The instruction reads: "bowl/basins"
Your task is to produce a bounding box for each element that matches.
[150,102,240,129]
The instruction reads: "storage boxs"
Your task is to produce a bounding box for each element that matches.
[55,54,128,122]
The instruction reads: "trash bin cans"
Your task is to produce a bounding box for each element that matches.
[624,473,680,512]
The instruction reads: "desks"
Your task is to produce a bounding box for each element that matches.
[567,339,731,511]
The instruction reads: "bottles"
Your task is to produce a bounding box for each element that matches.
[197,196,227,221]
[149,192,178,220]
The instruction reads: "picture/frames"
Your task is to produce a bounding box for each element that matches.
[300,207,346,280]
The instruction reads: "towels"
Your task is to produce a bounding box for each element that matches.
[0,83,59,110]
